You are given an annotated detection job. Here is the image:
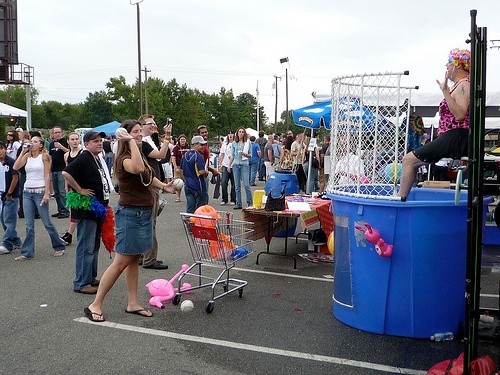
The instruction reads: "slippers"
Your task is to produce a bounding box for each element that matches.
[84,307,105,322]
[125,306,153,317]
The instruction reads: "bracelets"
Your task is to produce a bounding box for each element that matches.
[79,187,85,193]
[163,183,165,192]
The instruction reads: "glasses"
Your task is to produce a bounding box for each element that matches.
[141,122,156,126]
[6,134,14,136]
[448,58,453,63]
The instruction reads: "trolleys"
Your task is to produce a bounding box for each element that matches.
[171,212,255,313]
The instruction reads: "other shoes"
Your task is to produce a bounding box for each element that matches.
[53,250,64,256]
[247,175,265,187]
[52,211,70,218]
[14,255,34,261]
[175,197,181,202]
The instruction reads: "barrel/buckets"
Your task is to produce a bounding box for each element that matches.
[263,168,299,238]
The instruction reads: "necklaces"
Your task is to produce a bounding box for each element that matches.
[125,148,154,187]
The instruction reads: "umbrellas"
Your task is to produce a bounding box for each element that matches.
[291,95,397,134]
[84,121,126,138]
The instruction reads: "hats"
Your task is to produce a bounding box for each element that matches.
[451,49,470,72]
[191,136,208,145]
[84,130,107,142]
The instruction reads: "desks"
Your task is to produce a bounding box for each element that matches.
[245,193,331,269]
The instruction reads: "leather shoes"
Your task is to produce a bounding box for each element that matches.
[139,259,168,269]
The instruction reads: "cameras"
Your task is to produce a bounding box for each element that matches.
[167,118,173,125]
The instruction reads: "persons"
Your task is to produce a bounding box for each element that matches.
[85,118,181,322]
[394,49,469,197]
[62,131,112,294]
[136,113,172,269]
[0,124,388,264]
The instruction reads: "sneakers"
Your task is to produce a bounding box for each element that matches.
[229,201,236,205]
[60,232,72,246]
[0,246,9,253]
[74,279,100,294]
[232,204,242,209]
[14,245,22,249]
[220,201,228,206]
